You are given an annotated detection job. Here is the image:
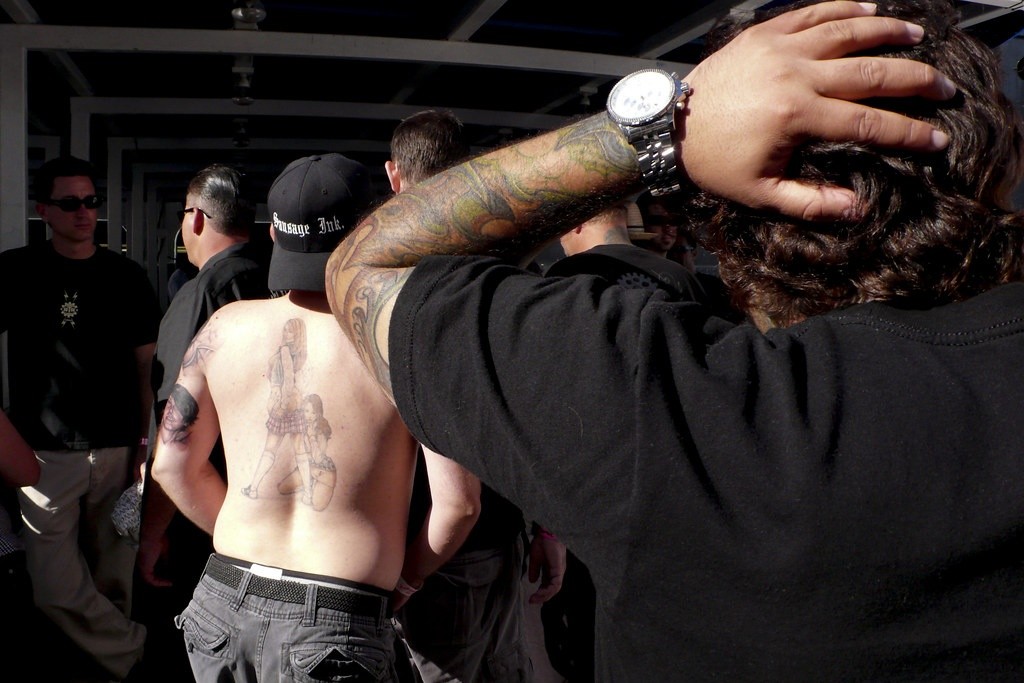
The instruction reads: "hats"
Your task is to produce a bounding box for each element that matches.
[624,200,660,240]
[266,153,373,291]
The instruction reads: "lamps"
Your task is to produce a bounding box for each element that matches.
[231,0,267,30]
[231,54,257,89]
[231,116,251,147]
[235,166,249,177]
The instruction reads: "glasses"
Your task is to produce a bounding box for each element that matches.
[176,207,211,224]
[38,194,104,212]
[648,214,679,227]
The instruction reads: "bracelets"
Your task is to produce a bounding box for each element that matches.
[538,525,562,544]
[396,576,424,596]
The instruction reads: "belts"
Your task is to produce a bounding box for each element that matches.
[206,556,395,619]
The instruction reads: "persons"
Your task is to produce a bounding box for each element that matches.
[0,109,748,683]
[323,0,1024,683]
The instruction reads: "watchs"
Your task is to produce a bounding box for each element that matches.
[607,68,692,196]
